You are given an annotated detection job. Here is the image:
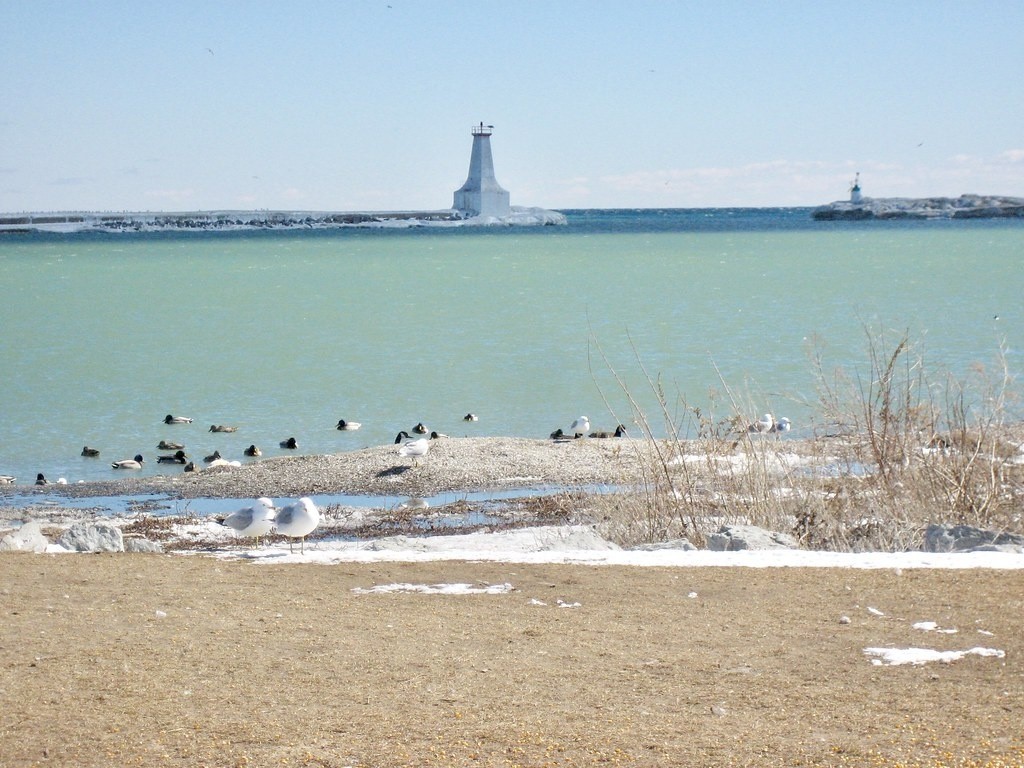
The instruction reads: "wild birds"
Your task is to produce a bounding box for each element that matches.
[464,413,479,422]
[35,473,50,485]
[397,498,429,511]
[184,462,200,473]
[335,420,361,431]
[588,424,626,438]
[162,414,193,424]
[394,431,428,467]
[244,445,262,456]
[747,413,792,442]
[550,428,575,439]
[279,438,298,449]
[274,498,319,555]
[214,497,276,552]
[571,415,590,439]
[157,441,185,450]
[412,423,429,435]
[156,451,188,464]
[429,432,450,440]
[0,475,18,486]
[202,451,221,464]
[81,447,100,458]
[112,454,146,469]
[208,424,239,433]
